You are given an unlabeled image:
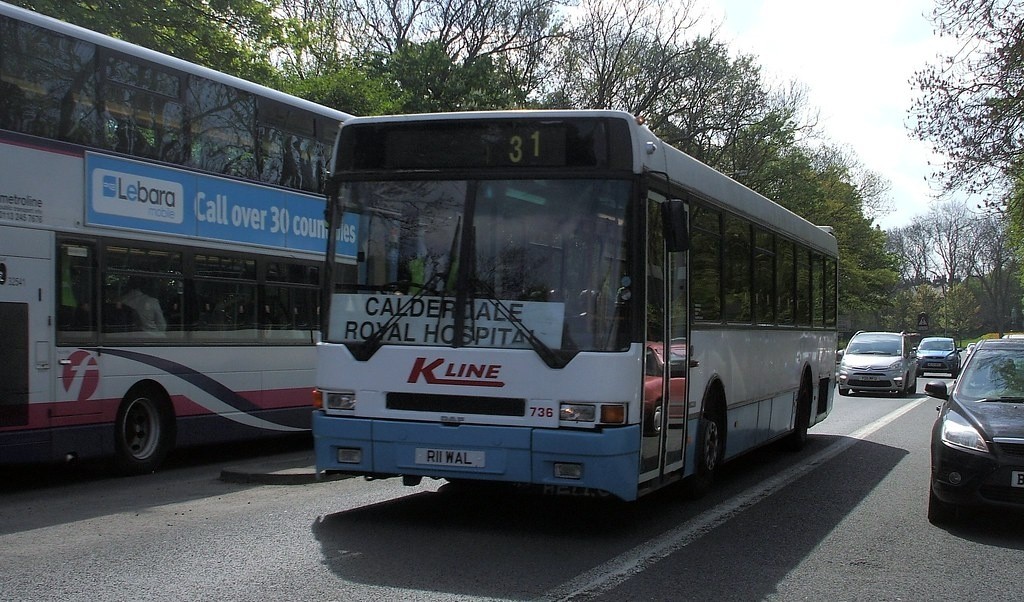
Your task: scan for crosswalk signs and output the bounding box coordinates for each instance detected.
[918,314,929,326]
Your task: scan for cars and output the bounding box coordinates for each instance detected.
[642,341,689,431]
[925,338,1024,527]
[836,331,919,394]
[914,338,961,378]
[965,342,975,355]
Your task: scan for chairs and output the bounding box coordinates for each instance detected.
[102,302,319,341]
[0,83,314,193]
[743,294,805,327]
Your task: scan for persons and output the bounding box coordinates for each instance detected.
[61,247,88,329]
[115,119,180,163]
[394,224,459,298]
[35,83,78,140]
[119,275,167,331]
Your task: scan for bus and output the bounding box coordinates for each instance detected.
[0,0,621,479]
[311,106,839,502]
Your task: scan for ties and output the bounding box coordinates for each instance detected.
[429,262,440,287]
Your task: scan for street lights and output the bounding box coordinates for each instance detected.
[934,281,947,336]
